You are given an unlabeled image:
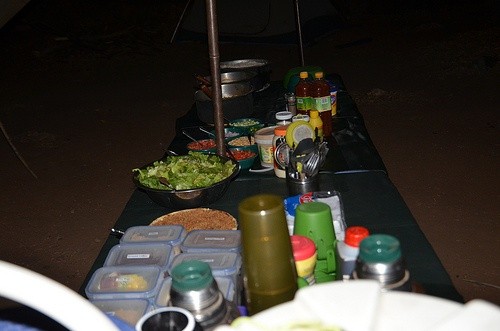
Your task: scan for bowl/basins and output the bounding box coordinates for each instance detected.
[131,152,241,208]
[185,118,262,169]
[86,226,243,326]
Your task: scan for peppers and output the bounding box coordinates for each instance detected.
[188,139,256,160]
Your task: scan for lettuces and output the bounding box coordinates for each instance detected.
[131,151,237,190]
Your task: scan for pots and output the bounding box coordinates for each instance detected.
[195,59,271,124]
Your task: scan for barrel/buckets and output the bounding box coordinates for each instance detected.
[254,126,278,168]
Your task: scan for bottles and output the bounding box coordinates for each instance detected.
[310,110,323,138]
[338,227,369,281]
[273,127,291,178]
[287,90,296,115]
[313,72,332,135]
[275,112,293,127]
[296,72,313,115]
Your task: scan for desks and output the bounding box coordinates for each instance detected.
[76,73,467,331]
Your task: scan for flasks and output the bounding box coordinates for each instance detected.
[166,260,240,331]
[350,235,414,292]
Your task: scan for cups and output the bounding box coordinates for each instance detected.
[238,194,296,295]
[329,90,337,117]
[292,203,337,283]
[288,173,319,196]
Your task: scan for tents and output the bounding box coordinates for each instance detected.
[169,0,342,49]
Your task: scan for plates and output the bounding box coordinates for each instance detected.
[148,208,238,230]
